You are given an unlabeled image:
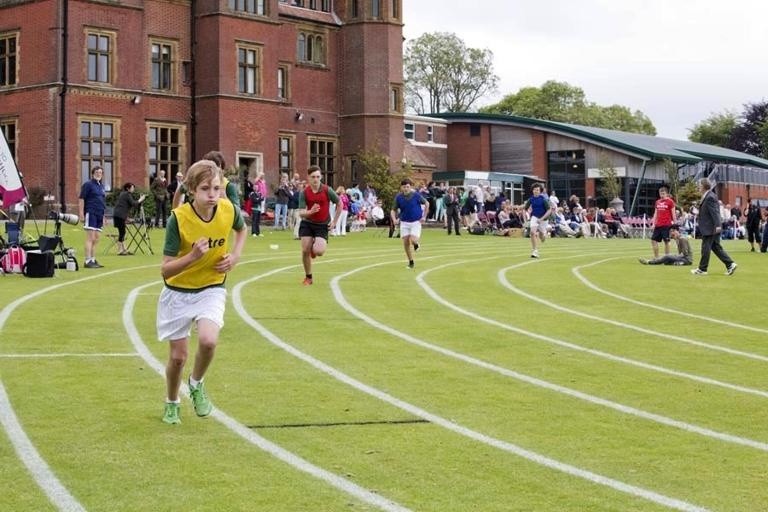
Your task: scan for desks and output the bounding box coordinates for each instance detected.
[125,222,154,255]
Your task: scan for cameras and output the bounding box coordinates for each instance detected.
[48,210,78,225]
[139,193,149,200]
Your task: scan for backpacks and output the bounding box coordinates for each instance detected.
[2,244,27,273]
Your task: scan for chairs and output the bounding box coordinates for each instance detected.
[476,210,655,235]
[103,220,119,255]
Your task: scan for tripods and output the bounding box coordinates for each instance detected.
[125,203,153,256]
[52,221,67,274]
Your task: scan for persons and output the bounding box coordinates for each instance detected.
[166,173,187,204]
[157,159,248,424]
[113,182,145,254]
[150,171,168,228]
[9,172,29,235]
[638,175,767,276]
[390,178,631,271]
[242,165,394,285]
[172,151,239,209]
[79,166,109,268]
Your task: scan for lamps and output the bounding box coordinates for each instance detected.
[131,96,141,104]
[295,113,303,119]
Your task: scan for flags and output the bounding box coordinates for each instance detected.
[0,127,27,211]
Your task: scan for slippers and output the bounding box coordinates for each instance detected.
[116,249,134,255]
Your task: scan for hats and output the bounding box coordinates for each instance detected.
[176,172,183,177]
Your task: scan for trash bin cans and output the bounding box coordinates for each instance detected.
[7,223,19,244]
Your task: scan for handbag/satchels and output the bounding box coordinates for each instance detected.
[22,250,54,278]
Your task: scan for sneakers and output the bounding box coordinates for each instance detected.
[414,243,419,252]
[690,268,707,275]
[303,277,312,286]
[408,260,414,268]
[92,260,104,268]
[638,255,649,265]
[188,374,212,416]
[84,260,99,268]
[162,397,180,424]
[531,249,538,259]
[724,263,737,276]
[310,252,316,258]
